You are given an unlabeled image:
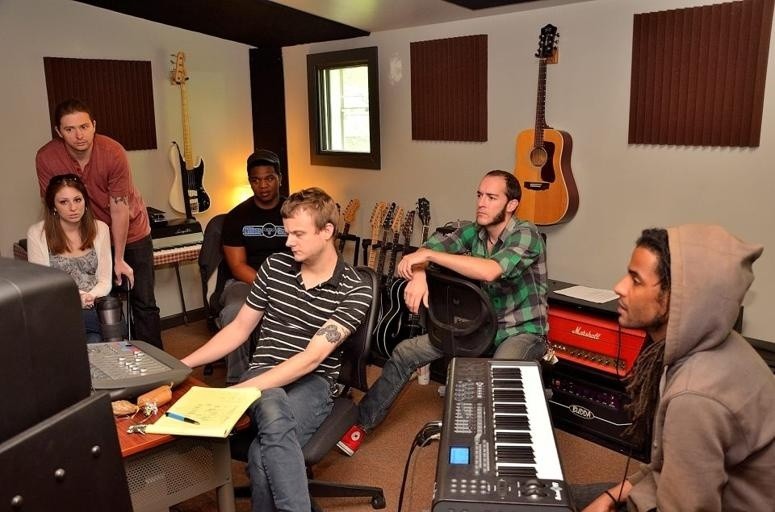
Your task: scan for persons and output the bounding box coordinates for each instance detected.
[181,187,372,510]
[578,223,775,512]
[27,98,161,353]
[218,150,295,431]
[335,170,549,457]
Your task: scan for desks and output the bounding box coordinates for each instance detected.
[112,375,251,512]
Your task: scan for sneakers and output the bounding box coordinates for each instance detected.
[336,423,366,458]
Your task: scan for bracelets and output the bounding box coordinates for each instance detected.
[603,488,619,506]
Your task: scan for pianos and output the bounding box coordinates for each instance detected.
[431,358,576,512]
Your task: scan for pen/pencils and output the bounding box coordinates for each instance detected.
[165,413,200,426]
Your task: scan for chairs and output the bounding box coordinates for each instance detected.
[414,268,498,446]
[112,247,137,340]
[232,266,386,512]
[198,213,233,376]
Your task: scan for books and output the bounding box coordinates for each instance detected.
[144,385,262,438]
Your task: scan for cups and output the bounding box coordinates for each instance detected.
[94,295,124,344]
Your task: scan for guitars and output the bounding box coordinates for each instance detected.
[338,197,431,360]
[513,24,579,226]
[169,50,210,214]
